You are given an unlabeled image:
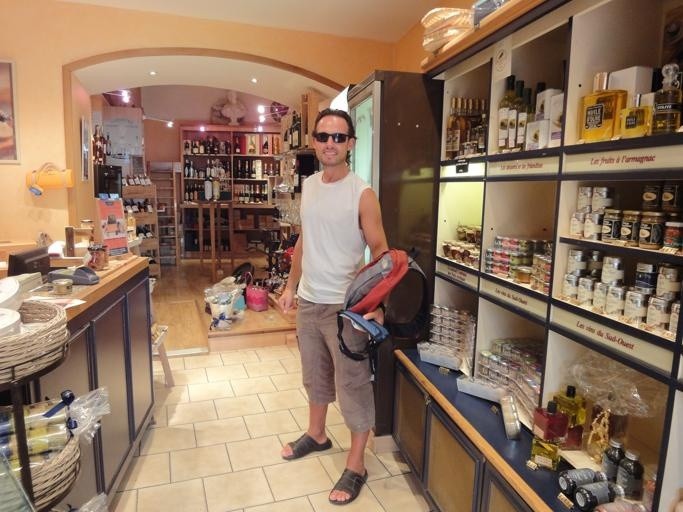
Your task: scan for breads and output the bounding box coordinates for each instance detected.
[0,401,73,432]
[0,425,74,454]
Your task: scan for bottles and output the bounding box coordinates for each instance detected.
[445,92,484,157]
[121,174,152,185]
[183,136,231,154]
[212,176,221,201]
[185,157,231,177]
[205,175,212,202]
[234,136,240,153]
[185,181,205,202]
[235,159,279,179]
[136,225,154,239]
[262,134,269,155]
[247,135,256,154]
[234,183,267,205]
[124,199,154,213]
[494,74,546,152]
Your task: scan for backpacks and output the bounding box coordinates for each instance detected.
[342,237,433,346]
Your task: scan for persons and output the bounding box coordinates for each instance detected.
[277,110,393,505]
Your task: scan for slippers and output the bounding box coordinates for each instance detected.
[329,466,368,506]
[282,435,332,461]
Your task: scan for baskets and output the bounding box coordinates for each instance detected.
[0,433,82,509]
[0,298,69,387]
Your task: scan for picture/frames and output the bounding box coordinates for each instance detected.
[0,60,22,165]
[80,115,90,182]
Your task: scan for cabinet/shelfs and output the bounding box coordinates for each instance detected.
[30,323,102,512]
[122,183,162,279]
[431,0,683,512]
[319,69,444,438]
[0,298,83,512]
[179,124,285,259]
[390,363,534,511]
[90,276,154,496]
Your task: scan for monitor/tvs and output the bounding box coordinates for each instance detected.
[93,164,122,197]
[7,246,51,276]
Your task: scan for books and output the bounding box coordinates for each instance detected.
[96,198,128,249]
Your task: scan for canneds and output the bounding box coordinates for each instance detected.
[430,298,477,370]
[601,209,619,243]
[663,221,682,250]
[639,212,664,249]
[486,235,550,294]
[441,222,480,268]
[620,209,641,247]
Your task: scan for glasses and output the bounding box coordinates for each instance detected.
[311,130,357,145]
